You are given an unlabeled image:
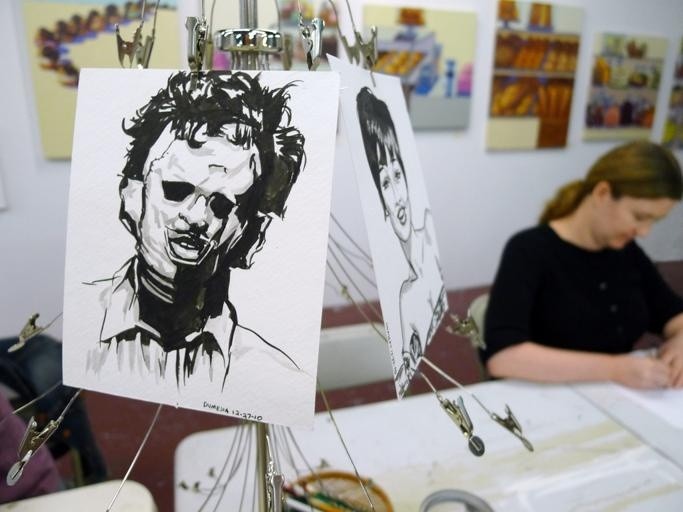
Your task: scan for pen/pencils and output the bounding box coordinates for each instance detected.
[281,481,375,512]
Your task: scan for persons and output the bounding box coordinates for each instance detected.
[357,86,450,359]
[481,139,683,392]
[85,73,301,410]
